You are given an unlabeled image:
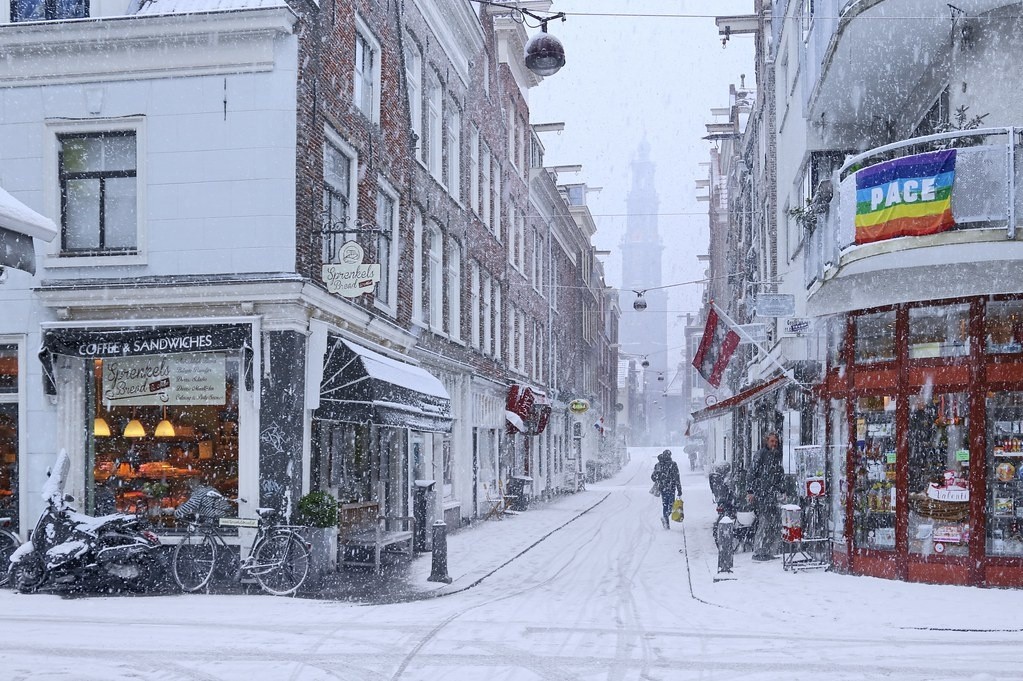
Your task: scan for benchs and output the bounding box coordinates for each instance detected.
[483,478,519,522]
[338,498,417,575]
[564,471,586,494]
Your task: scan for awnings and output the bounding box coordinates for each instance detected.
[686,429,706,439]
[509,385,551,435]
[314,336,452,434]
[691,375,794,423]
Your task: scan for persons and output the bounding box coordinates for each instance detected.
[100,476,122,515]
[649,450,682,529]
[174,475,233,527]
[688,452,697,471]
[747,431,783,561]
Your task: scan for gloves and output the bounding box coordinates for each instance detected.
[677,491,682,495]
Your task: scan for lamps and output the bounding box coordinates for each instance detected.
[94,374,111,437]
[632,289,647,312]
[123,406,146,437]
[522,7,567,76]
[641,354,649,368]
[154,405,176,437]
[657,371,664,383]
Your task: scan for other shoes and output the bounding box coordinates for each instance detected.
[764,554,780,560]
[661,516,670,529]
[752,555,767,561]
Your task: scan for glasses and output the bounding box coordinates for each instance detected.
[769,432,779,437]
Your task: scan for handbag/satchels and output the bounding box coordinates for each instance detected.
[649,482,659,497]
[672,496,684,522]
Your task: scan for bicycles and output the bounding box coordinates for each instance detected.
[711,491,756,553]
[1,518,22,587]
[172,478,313,598]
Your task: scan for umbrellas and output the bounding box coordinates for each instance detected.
[683,444,700,453]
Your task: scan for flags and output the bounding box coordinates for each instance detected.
[594,417,605,437]
[853,148,958,244]
[692,309,740,388]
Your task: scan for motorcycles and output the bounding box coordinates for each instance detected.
[8,445,167,596]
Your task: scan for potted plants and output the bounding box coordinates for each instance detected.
[289,489,339,579]
